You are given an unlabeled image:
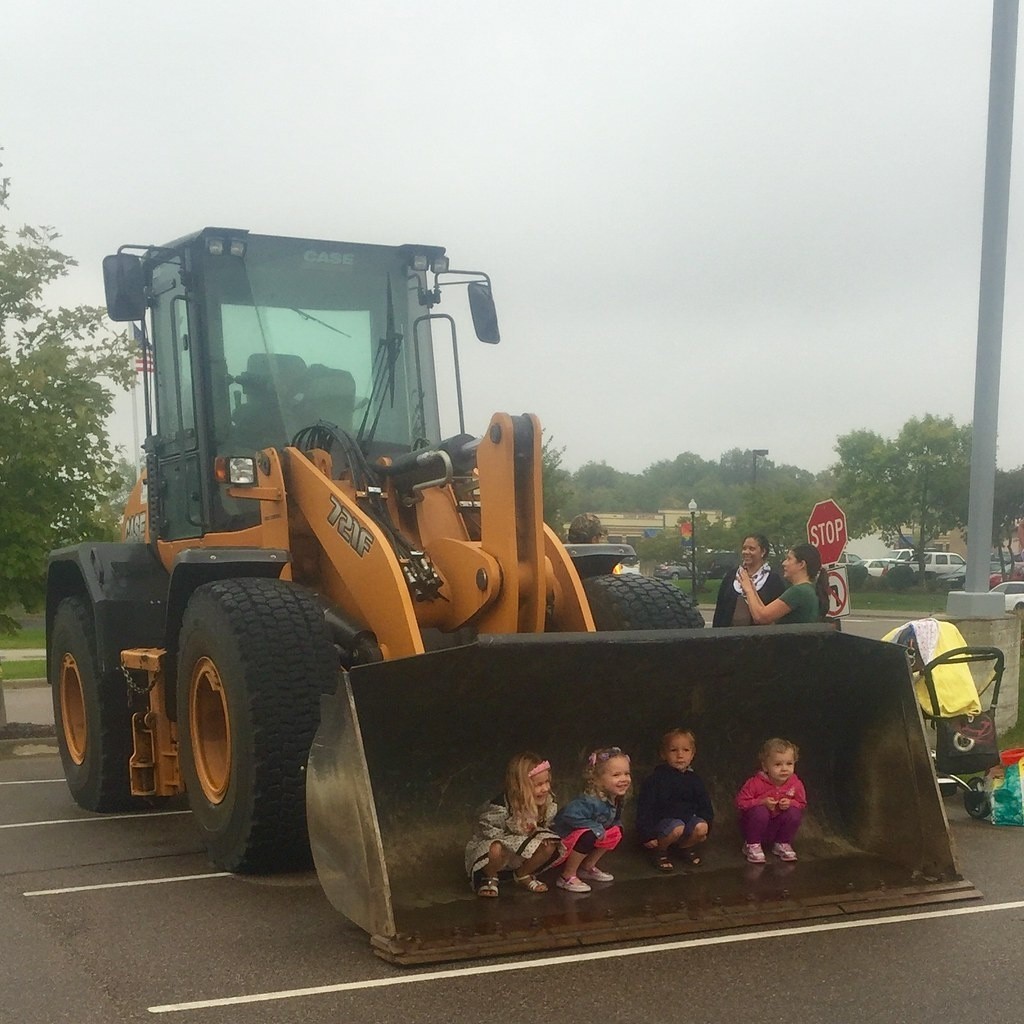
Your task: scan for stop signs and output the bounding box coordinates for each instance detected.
[805,499,849,565]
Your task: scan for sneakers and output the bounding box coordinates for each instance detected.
[742,840,766,863]
[575,865,613,881]
[556,875,590,892]
[771,841,798,861]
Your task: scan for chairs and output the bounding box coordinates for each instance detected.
[235,353,306,449]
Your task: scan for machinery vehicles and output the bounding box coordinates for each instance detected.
[41,225,986,964]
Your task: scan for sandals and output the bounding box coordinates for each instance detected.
[477,877,499,897]
[676,848,702,867]
[514,869,549,892]
[652,850,674,874]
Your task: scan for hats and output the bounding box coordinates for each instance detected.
[568,514,608,542]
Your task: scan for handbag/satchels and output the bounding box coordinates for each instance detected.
[924,656,1003,774]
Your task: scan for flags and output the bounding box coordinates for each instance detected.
[133,323,155,371]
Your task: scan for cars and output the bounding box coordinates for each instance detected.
[851,559,903,577]
[991,561,1024,590]
[653,561,698,581]
[843,554,862,565]
[991,581,1024,612]
[886,548,917,562]
[903,552,967,574]
[935,562,1007,591]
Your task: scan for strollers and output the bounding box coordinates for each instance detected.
[895,622,1005,820]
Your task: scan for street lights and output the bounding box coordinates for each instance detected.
[751,448,769,490]
[687,497,703,606]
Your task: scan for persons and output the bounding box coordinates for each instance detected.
[732,738,809,863]
[711,533,787,627]
[633,728,716,873]
[461,752,566,898]
[544,747,631,893]
[568,513,621,576]
[736,543,830,623]
